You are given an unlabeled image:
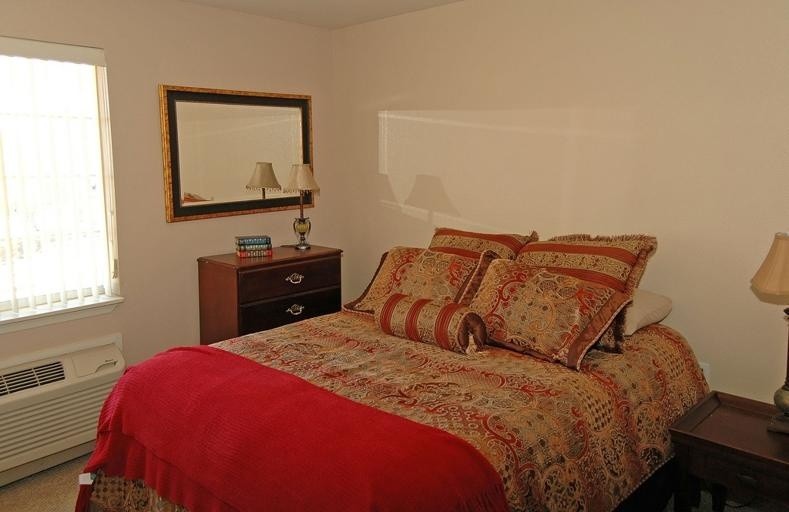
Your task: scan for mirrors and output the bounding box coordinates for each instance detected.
[158,84,314,223]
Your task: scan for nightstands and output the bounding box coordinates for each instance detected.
[667,391,789,512]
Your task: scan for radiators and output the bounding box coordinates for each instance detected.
[0,329,127,490]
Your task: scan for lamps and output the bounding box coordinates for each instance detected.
[284,157,321,251]
[749,232,789,442]
[247,161,282,199]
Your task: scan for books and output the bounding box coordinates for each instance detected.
[236,257,272,266]
[234,235,273,257]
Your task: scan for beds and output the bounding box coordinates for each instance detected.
[76,305,711,512]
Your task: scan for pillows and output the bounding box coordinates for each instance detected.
[345,248,493,321]
[433,230,541,263]
[626,290,675,338]
[378,292,489,357]
[456,249,636,372]
[515,236,657,355]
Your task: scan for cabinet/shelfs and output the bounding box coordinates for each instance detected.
[197,244,345,345]
